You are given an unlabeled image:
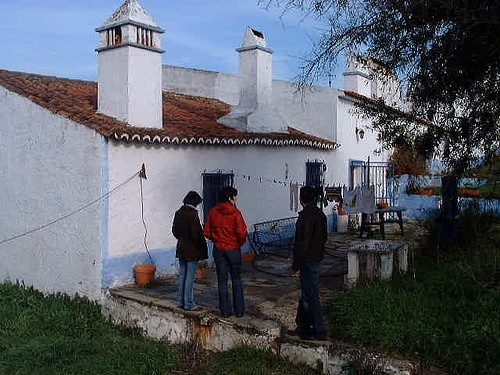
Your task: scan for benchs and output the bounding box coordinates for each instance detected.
[252,216,299,258]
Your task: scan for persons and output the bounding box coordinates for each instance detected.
[203,186,248,318]
[172,191,208,311]
[287,186,328,341]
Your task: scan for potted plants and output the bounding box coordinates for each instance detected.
[195,262,209,279]
[458,183,480,197]
[405,180,433,196]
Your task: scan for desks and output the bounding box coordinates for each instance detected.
[359,206,406,240]
[345,240,407,286]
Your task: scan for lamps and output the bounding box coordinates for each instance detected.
[355,127,365,139]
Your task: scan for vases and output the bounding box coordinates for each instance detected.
[135,262,156,286]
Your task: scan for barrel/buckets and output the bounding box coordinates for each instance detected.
[134,263,156,288]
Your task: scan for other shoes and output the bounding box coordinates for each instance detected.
[221,311,233,318]
[237,311,244,318]
[302,334,327,341]
[287,328,299,336]
[184,304,203,311]
